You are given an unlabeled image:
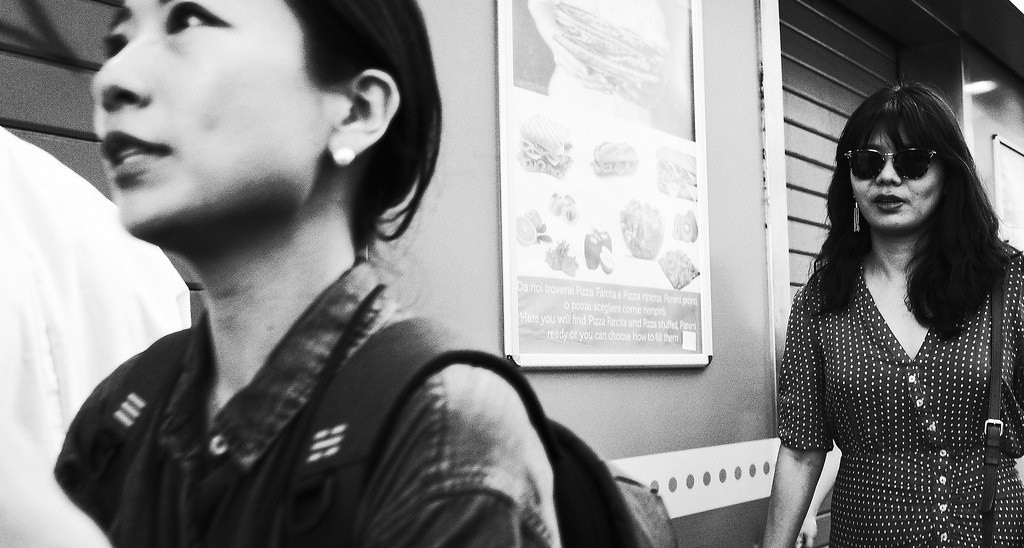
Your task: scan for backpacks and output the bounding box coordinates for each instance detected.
[80,314,679,547]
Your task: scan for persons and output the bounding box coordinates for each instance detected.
[761,84,1024,548]
[0,0,563,547]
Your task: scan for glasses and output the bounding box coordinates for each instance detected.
[843,148,938,180]
